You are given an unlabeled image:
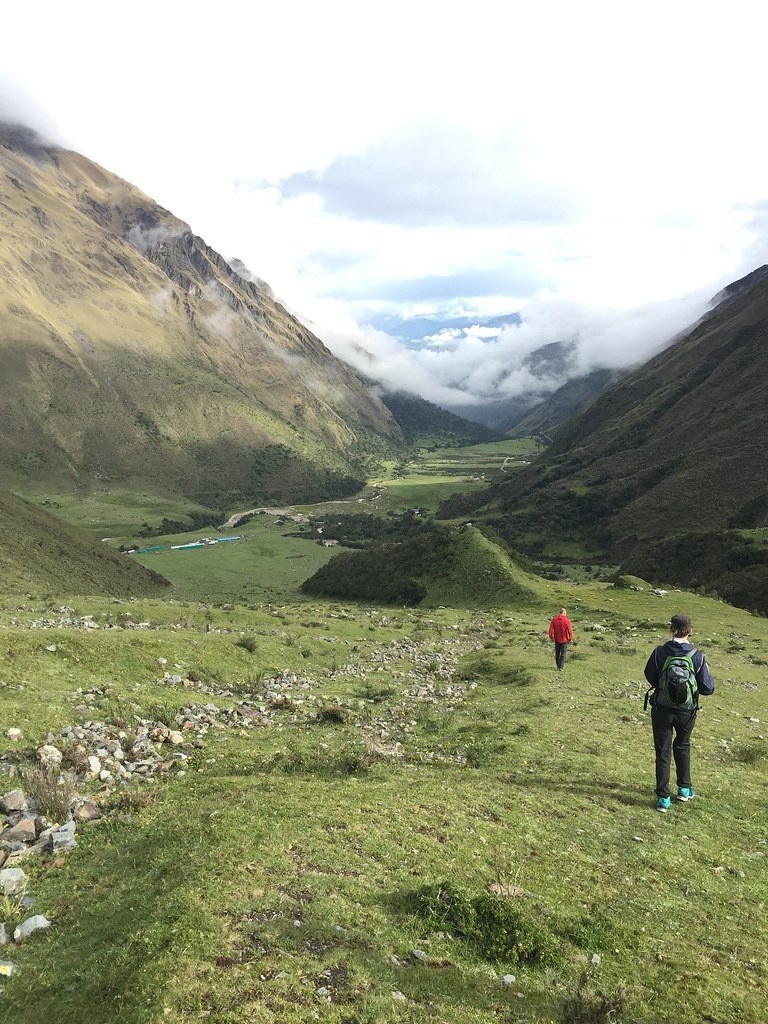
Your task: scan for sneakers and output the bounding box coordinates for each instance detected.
[656,796,671,812]
[677,787,694,801]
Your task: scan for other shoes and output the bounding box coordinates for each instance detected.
[559,668,563,671]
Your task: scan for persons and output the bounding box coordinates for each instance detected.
[644,615,715,813]
[549,608,572,672]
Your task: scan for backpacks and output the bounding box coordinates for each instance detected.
[654,646,698,711]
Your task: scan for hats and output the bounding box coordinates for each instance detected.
[671,614,692,630]
